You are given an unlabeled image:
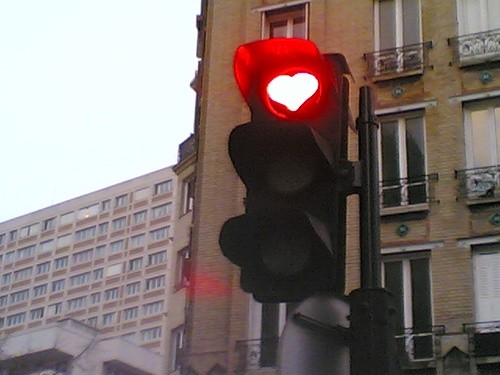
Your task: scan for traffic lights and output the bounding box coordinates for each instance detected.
[219,37,354,302]
